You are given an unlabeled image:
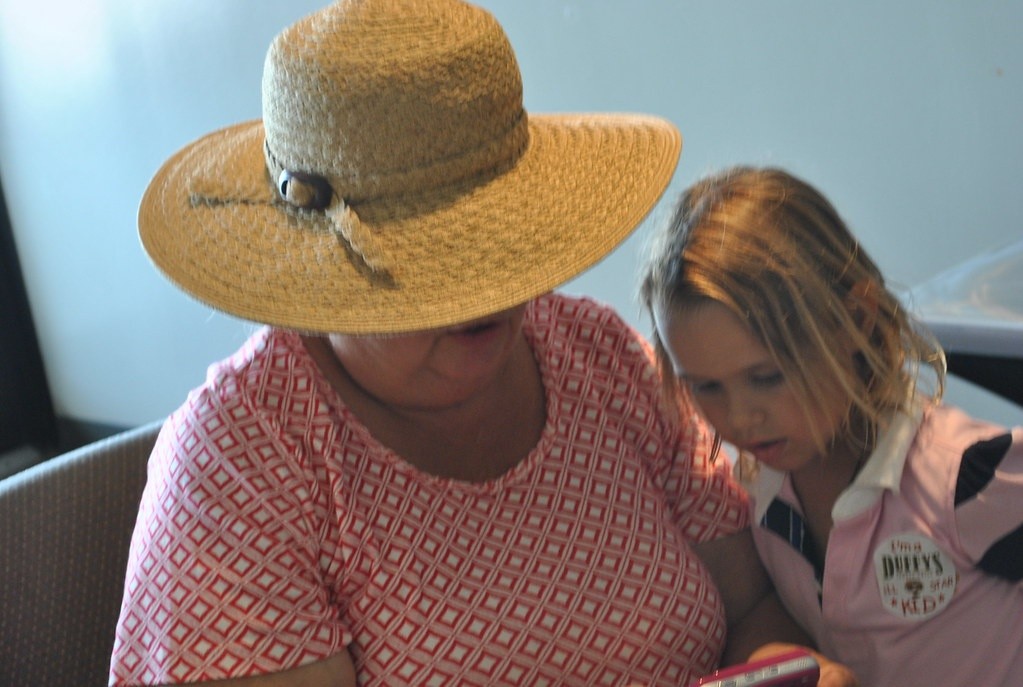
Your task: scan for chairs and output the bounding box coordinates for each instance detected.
[0,419,164,687]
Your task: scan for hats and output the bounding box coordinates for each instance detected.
[137,0,683,335]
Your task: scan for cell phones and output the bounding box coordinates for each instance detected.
[691,650,820,687]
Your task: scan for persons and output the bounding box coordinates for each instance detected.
[641,164,1023,687]
[106,1,855,687]
